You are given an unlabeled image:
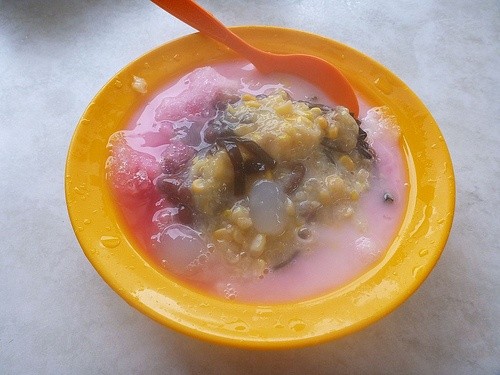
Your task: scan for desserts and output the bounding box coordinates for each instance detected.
[106,56,410,306]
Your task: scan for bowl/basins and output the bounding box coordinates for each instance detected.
[63,25,457,347]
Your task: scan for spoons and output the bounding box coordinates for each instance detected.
[149,0,361,121]
